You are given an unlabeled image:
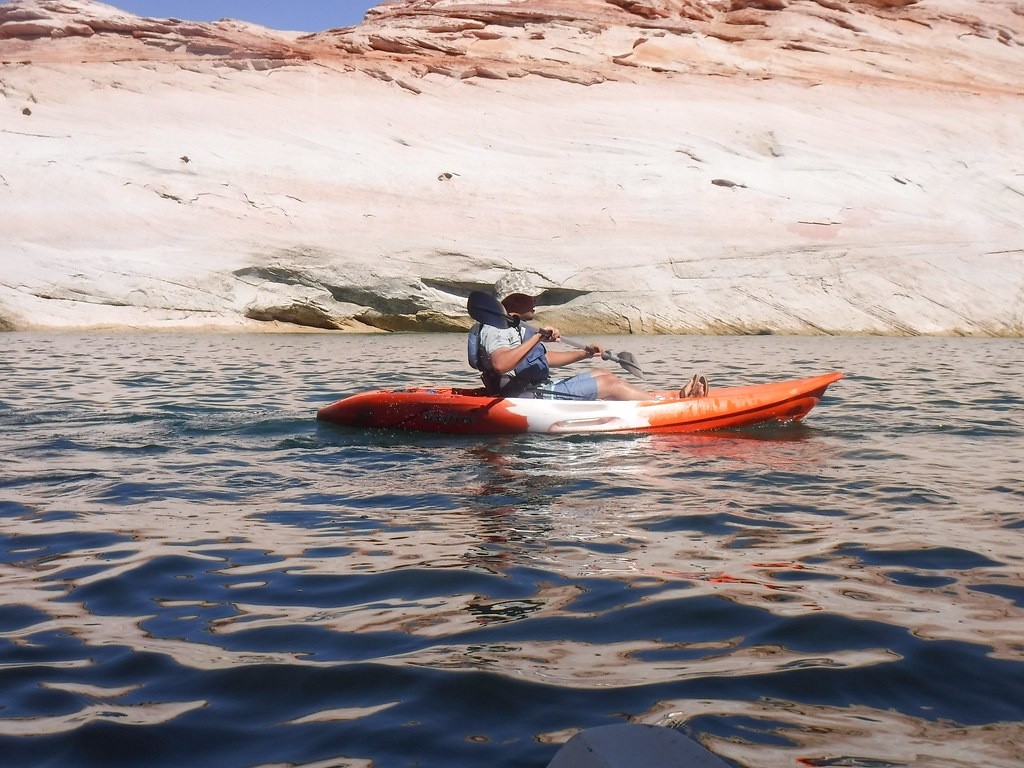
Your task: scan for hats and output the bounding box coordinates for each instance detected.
[494,271,548,302]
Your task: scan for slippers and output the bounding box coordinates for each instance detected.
[688,375,709,398]
[679,374,699,398]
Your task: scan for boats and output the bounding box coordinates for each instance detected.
[314,371,845,434]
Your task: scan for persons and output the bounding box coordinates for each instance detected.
[478,272,709,401]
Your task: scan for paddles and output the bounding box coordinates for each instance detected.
[467,290,645,382]
